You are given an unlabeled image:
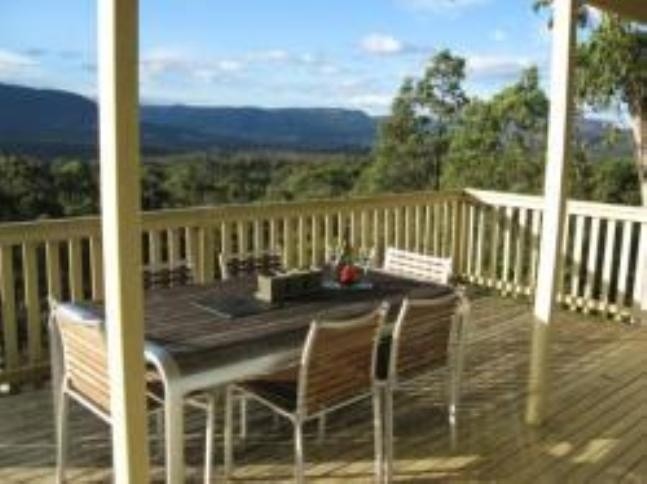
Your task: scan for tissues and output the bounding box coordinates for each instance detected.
[257,266,325,301]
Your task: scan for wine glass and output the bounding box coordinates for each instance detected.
[359,247,375,284]
